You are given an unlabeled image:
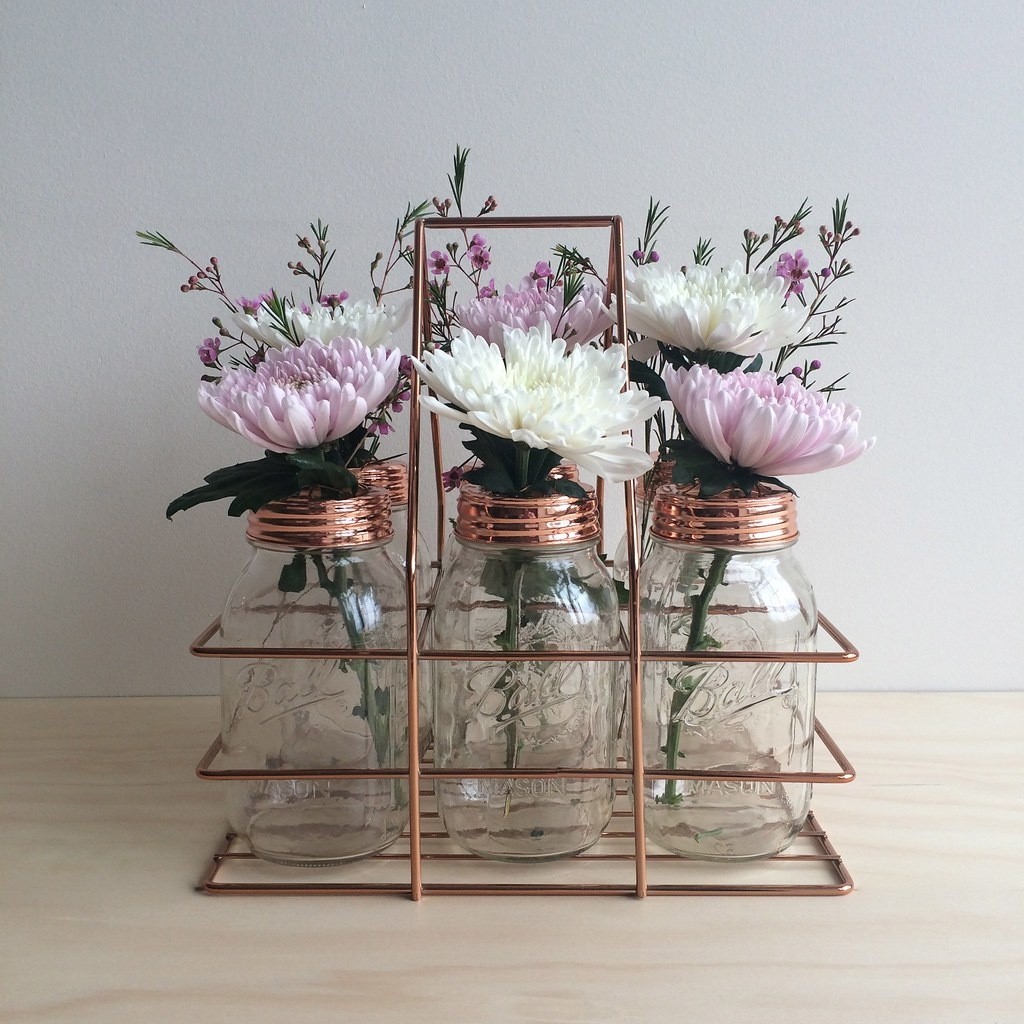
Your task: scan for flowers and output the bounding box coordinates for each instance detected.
[135,141,876,805]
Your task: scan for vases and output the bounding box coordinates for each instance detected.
[208,450,825,866]
